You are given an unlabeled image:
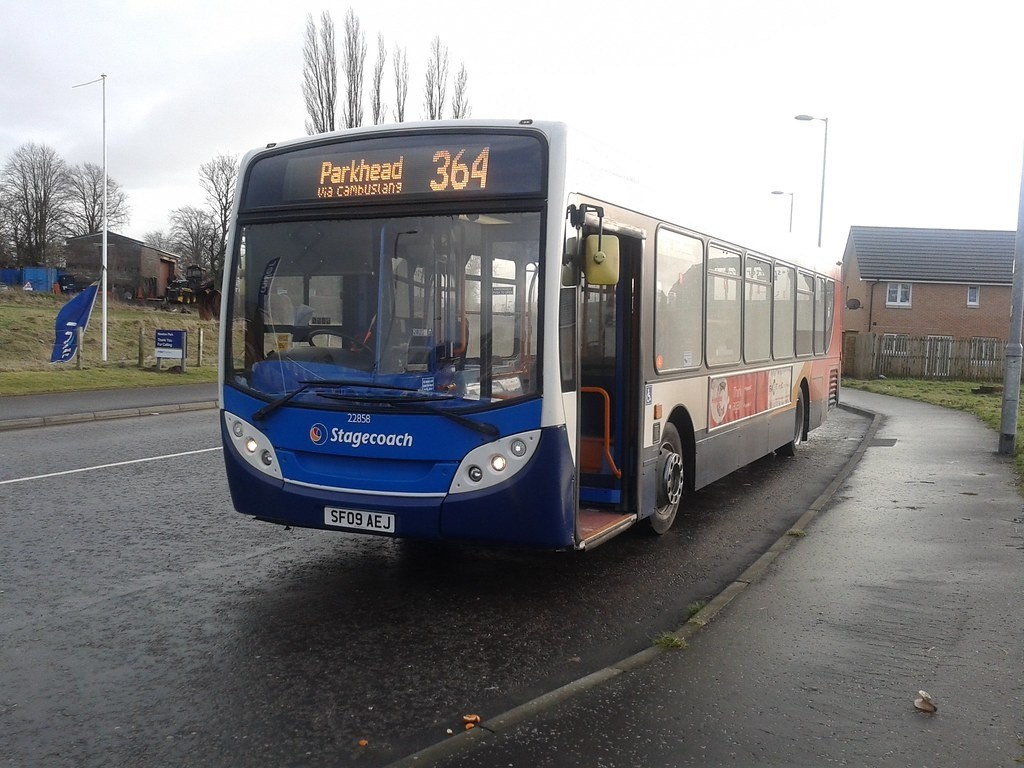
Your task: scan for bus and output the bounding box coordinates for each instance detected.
[217,118,845,556]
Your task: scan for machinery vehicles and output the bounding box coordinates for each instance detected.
[167,264,207,305]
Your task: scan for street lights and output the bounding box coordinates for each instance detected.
[794,114,828,249]
[771,190,794,233]
[394,229,418,290]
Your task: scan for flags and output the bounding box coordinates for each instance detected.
[49,278,101,365]
